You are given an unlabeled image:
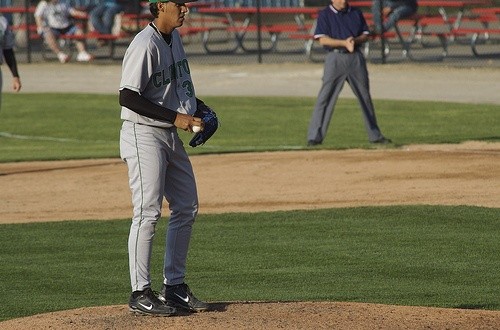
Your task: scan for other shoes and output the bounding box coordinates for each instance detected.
[372,137,392,143]
[57,51,69,64]
[76,50,94,62]
[306,139,321,146]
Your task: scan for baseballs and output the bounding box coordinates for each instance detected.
[191,125,202,133]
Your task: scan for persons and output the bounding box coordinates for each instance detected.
[307,0,420,148]
[34,0,129,64]
[0,10,21,108]
[119,0,215,316]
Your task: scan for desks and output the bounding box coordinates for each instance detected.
[0,7,42,13]
[196,7,327,55]
[348,1,491,42]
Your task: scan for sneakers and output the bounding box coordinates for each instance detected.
[158,281,210,313]
[127,287,177,317]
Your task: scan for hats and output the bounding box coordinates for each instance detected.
[149,0,198,4]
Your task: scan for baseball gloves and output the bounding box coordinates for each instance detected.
[184,97,220,149]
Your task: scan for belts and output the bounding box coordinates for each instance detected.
[331,48,349,53]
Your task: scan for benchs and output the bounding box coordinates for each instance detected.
[10,17,500,62]
